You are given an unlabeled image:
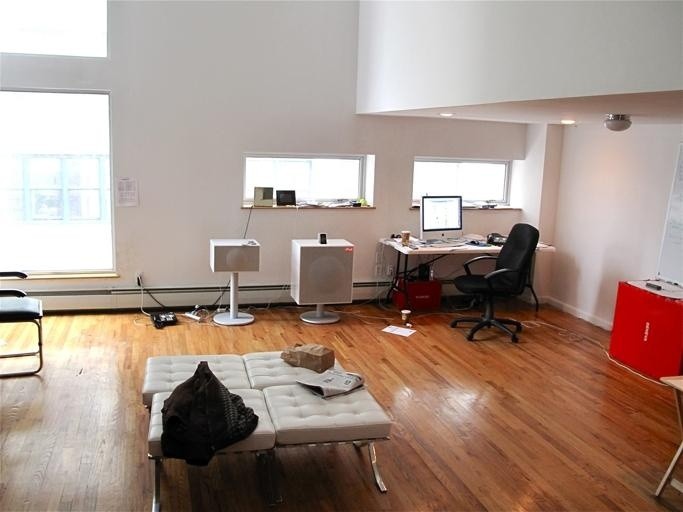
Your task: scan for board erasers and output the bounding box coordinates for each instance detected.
[646,283,662,291]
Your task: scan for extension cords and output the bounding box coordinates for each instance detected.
[184,312,203,322]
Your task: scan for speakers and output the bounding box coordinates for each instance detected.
[290,233,355,306]
[209,237,261,273]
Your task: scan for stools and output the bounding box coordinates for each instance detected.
[264,384,392,501]
[144,354,250,390]
[244,350,347,389]
[149,392,275,511]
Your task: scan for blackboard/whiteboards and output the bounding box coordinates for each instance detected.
[656,143,683,289]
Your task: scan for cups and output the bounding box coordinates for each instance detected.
[402,231,410,247]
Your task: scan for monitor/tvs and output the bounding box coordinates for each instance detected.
[420,195,465,247]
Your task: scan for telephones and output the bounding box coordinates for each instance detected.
[487,232,508,245]
[150,310,177,328]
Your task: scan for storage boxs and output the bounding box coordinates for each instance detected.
[394,278,442,312]
[611,278,683,385]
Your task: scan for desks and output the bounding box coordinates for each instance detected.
[654,376,683,501]
[379,237,556,312]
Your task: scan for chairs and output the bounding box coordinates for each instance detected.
[455,223,539,341]
[0,272,44,379]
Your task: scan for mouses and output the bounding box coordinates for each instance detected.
[470,240,479,245]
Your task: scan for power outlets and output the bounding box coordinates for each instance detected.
[135,272,145,287]
